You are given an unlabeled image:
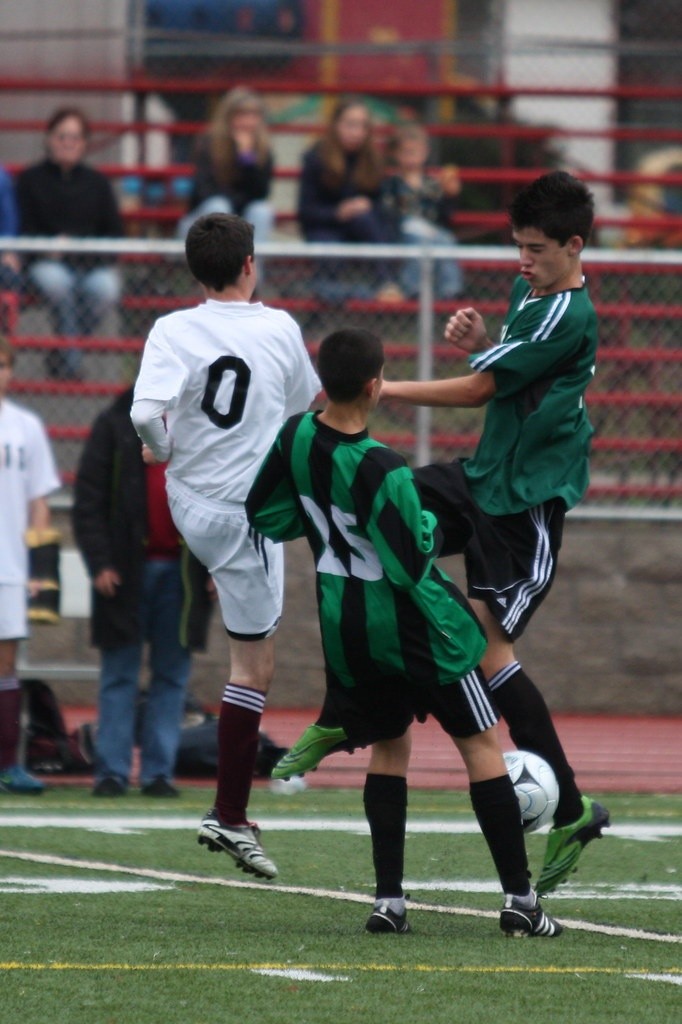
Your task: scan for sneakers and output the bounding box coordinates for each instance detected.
[197,807,279,879]
[0,766,44,794]
[500,888,564,939]
[272,724,354,781]
[534,795,610,897]
[364,891,412,935]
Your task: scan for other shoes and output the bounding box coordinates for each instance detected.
[92,778,125,796]
[141,776,180,798]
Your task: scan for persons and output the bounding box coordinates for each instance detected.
[245,331,564,936]
[70,385,217,799]
[0,338,62,796]
[0,167,23,339]
[131,211,322,880]
[270,169,612,899]
[297,100,474,303]
[177,86,274,297]
[11,106,126,384]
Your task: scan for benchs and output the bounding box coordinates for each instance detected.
[0,63,682,499]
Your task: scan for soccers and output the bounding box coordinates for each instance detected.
[501,748,562,835]
